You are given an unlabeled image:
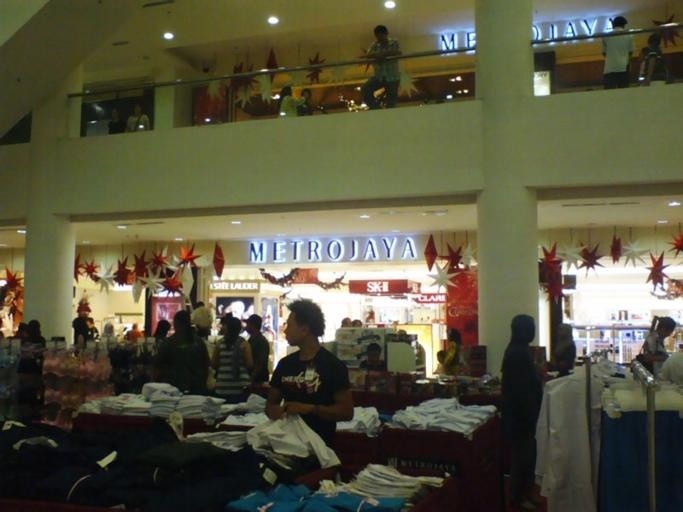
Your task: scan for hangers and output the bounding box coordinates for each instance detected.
[0,334,161,362]
[601,360,682,420]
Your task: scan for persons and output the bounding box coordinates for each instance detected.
[361,25,402,109]
[360,343,385,370]
[108,102,150,134]
[432,329,461,374]
[152,301,270,403]
[642,316,676,361]
[279,85,312,117]
[501,315,576,512]
[126,323,143,340]
[601,16,666,89]
[72,318,99,357]
[266,299,353,447]
[16,320,45,403]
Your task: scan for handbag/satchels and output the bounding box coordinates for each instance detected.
[636,334,658,374]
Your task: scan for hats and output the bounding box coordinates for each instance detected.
[243,315,263,328]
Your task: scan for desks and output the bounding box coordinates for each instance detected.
[70,373,507,512]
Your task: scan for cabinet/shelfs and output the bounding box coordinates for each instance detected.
[323,324,425,382]
